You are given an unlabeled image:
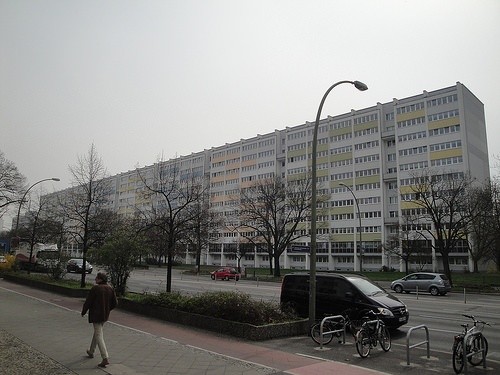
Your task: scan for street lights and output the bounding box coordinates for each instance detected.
[308,80,370,338]
[338,182,363,272]
[13,177,61,247]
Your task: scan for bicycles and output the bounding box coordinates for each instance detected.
[309,308,357,346]
[352,309,392,359]
[451,314,492,374]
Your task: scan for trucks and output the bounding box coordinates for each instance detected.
[18,241,61,273]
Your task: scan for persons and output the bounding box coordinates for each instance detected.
[81,272,118,366]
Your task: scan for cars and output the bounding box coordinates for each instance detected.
[390,272,452,297]
[209,267,242,282]
[66,259,94,274]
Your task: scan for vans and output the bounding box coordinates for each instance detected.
[279,271,410,331]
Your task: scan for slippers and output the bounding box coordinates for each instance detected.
[86,349,94,358]
[98,361,109,367]
